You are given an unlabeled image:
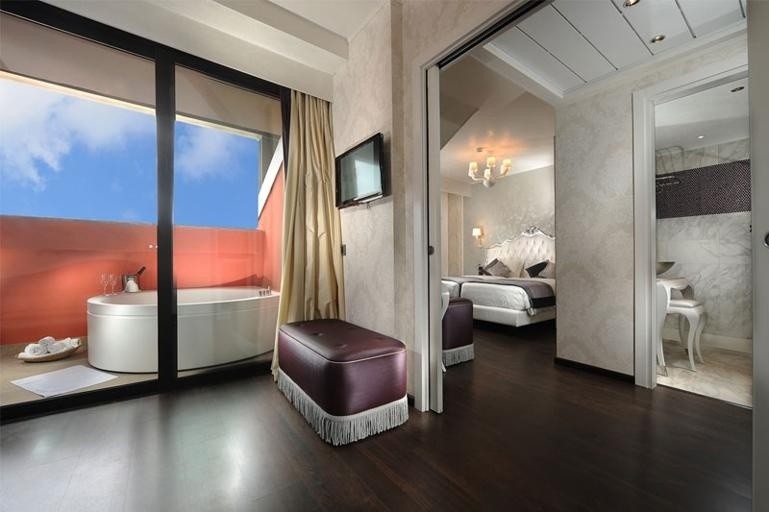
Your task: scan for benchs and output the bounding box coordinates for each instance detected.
[271,318,409,447]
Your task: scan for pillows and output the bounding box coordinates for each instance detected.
[479,253,556,277]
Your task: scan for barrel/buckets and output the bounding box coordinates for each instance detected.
[122,271,139,291]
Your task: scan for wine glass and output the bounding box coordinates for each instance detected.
[110,271,118,296]
[98,271,110,297]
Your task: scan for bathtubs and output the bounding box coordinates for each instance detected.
[87,286,280,374]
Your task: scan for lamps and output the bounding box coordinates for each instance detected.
[467,152,512,189]
[472,227,483,249]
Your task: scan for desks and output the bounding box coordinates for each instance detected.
[655,276,694,376]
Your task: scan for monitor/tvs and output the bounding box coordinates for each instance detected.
[333,132,386,208]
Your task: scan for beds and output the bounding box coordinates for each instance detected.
[440,227,557,328]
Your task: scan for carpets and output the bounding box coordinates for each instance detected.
[9,365,119,398]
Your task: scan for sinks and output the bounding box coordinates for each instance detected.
[655,260,676,275]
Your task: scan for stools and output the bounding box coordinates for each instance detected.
[666,298,708,372]
